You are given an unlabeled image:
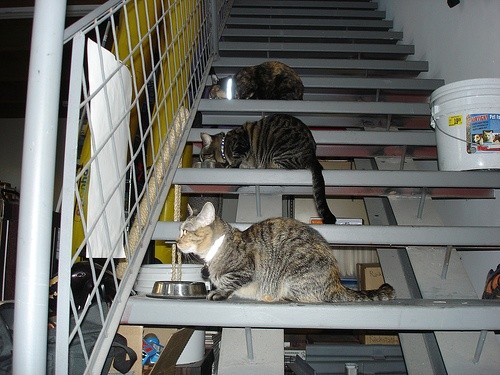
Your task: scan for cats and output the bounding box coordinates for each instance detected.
[175,202,396,303]
[200,113,336,224]
[483,130,500,143]
[209,61,305,101]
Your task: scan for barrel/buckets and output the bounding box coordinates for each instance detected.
[425,76,500,172]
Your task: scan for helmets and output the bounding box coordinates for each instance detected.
[47,261,137,315]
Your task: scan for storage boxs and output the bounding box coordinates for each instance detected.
[107,324,196,375]
[355,262,401,345]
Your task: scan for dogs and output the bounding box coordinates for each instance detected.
[473,134,484,145]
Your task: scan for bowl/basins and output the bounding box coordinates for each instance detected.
[146,280,208,299]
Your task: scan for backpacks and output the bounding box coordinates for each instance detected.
[0,302,137,375]
